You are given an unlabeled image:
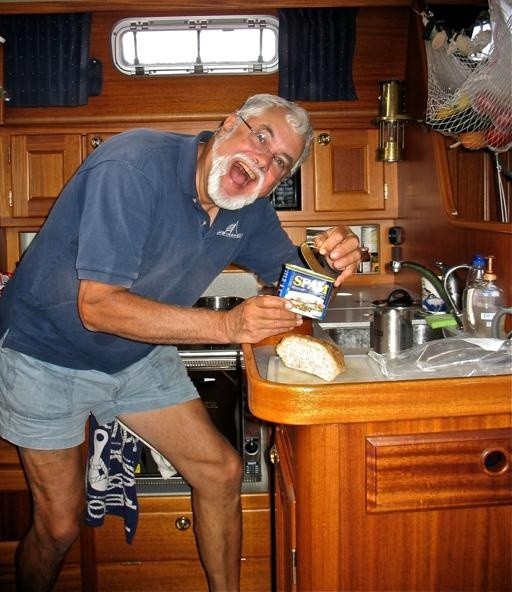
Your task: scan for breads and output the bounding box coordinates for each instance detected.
[277,335,346,381]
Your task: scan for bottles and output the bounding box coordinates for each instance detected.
[357,247,371,273]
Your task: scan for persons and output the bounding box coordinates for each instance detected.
[0,92,362,591]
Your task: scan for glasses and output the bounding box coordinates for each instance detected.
[239,115,288,172]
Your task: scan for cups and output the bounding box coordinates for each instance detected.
[375,306,415,354]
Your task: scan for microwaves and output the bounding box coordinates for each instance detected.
[134,357,269,496]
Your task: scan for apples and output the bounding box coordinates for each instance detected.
[472,91,512,147]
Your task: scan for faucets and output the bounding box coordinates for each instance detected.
[389,258,460,313]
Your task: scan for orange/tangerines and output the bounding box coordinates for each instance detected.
[462,132,485,148]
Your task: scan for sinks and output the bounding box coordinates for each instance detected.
[320,318,476,360]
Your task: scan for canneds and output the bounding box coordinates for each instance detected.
[276,264,335,321]
[357,227,379,273]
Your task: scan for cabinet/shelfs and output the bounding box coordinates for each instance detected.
[1,115,512,592]
